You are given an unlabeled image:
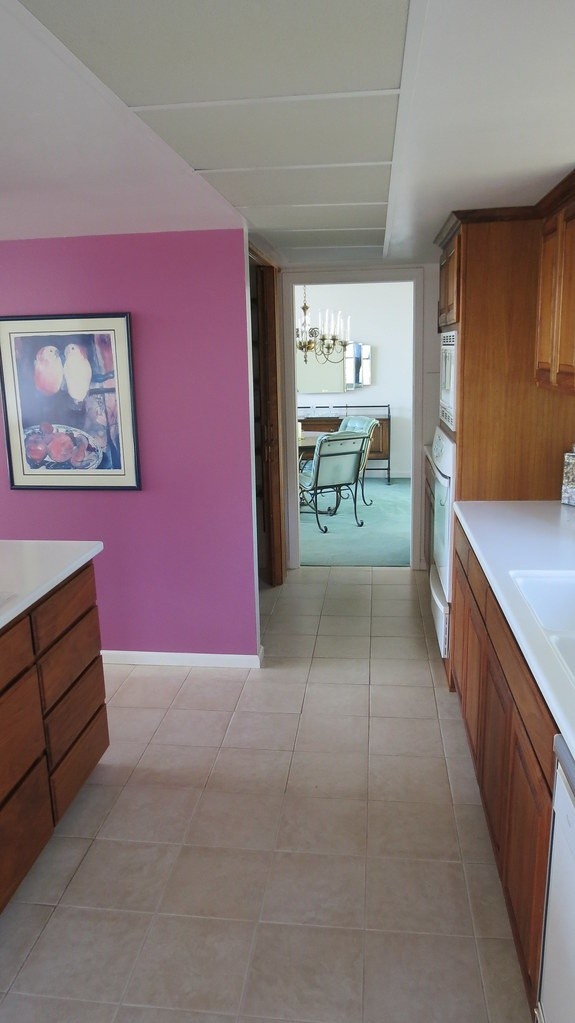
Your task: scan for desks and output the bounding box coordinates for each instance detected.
[298,430,331,513]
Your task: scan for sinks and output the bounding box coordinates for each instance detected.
[509,564,571,677]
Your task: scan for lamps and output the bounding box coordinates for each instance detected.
[296,285,350,363]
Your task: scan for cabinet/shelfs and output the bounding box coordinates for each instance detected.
[445,519,559,1023]
[0,539,110,911]
[535,203,575,398]
[437,234,460,327]
[298,406,391,484]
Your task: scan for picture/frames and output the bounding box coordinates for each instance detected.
[0,310,141,490]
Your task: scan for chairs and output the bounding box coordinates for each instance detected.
[299,430,369,533]
[328,415,380,510]
